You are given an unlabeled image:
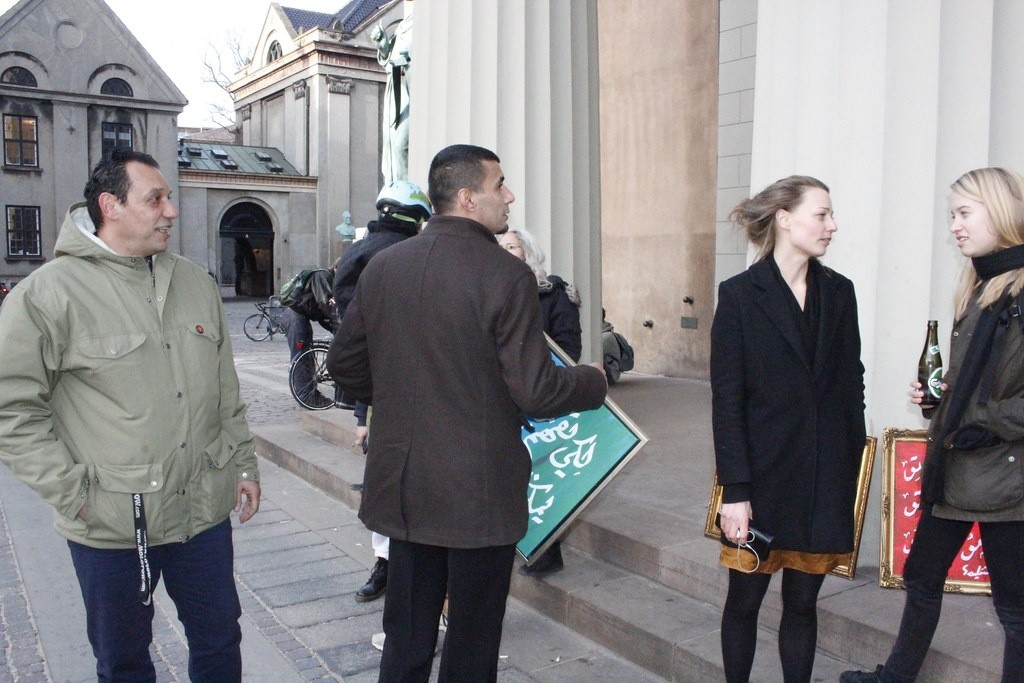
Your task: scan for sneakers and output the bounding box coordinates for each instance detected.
[439,613,449,630]
[839,664,884,683]
[372,633,439,657]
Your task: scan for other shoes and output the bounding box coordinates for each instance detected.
[300,390,333,409]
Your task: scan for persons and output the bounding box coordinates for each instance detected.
[275,252,348,408]
[371,11,414,187]
[325,145,635,683]
[839,167,1024,683]
[708,175,866,683]
[335,210,356,236]
[1,146,264,683]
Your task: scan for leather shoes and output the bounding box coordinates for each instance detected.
[355,557,389,602]
[518,541,564,577]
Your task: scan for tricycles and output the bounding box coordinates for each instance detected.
[243,295,287,342]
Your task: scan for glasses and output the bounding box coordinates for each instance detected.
[499,243,522,250]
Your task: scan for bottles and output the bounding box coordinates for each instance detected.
[917,319,942,406]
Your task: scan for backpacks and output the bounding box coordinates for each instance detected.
[278,268,334,307]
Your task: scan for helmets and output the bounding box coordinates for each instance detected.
[376,181,432,221]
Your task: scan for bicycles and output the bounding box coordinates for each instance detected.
[288,339,337,412]
